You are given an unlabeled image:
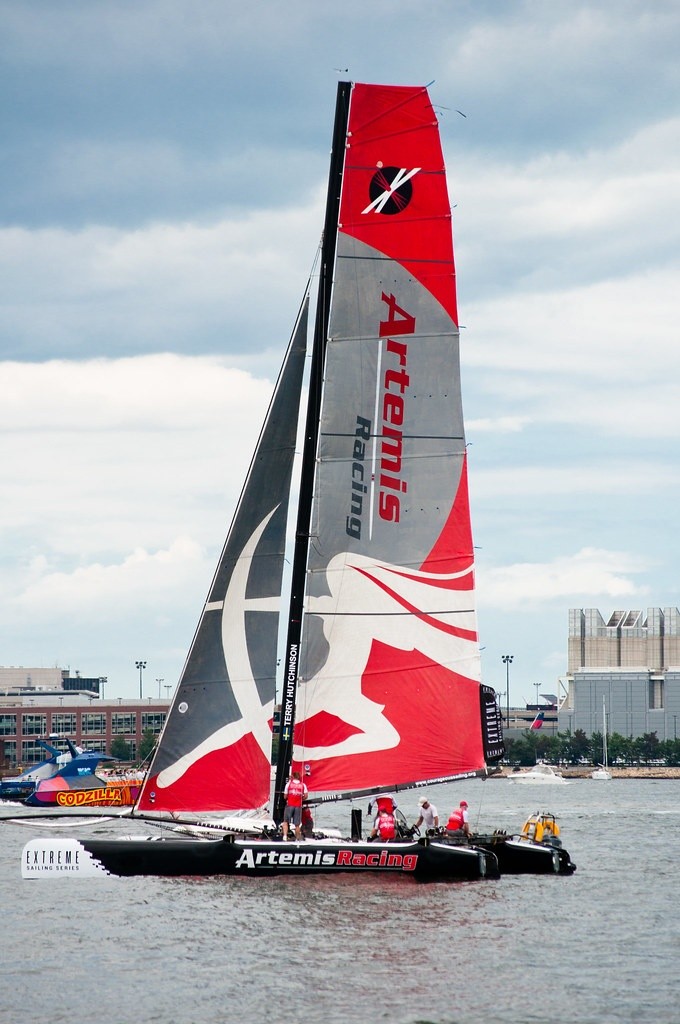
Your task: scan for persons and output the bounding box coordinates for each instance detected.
[21,775,39,787]
[283,772,308,841]
[368,793,397,837]
[76,745,83,754]
[445,801,468,836]
[373,809,396,841]
[104,769,146,780]
[416,797,438,829]
[565,764,568,769]
[301,808,313,837]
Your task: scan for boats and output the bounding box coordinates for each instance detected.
[501,831,575,876]
[23,739,149,806]
[506,761,572,787]
[1,711,84,799]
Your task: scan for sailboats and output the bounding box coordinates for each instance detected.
[74,80,501,880]
[592,692,612,780]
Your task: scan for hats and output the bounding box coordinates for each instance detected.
[417,797,427,807]
[460,801,468,807]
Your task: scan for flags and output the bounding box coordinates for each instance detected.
[531,712,545,729]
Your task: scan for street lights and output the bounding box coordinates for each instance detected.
[533,682,542,705]
[496,690,506,709]
[147,694,152,708]
[605,712,610,752]
[74,669,81,679]
[568,715,572,735]
[502,655,514,727]
[155,678,164,699]
[135,660,147,698]
[164,685,172,699]
[552,722,555,740]
[59,695,65,707]
[88,696,91,705]
[100,675,108,700]
[30,698,32,705]
[673,715,677,737]
[116,697,124,707]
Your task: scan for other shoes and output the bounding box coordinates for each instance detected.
[282,835,287,841]
[295,839,300,842]
